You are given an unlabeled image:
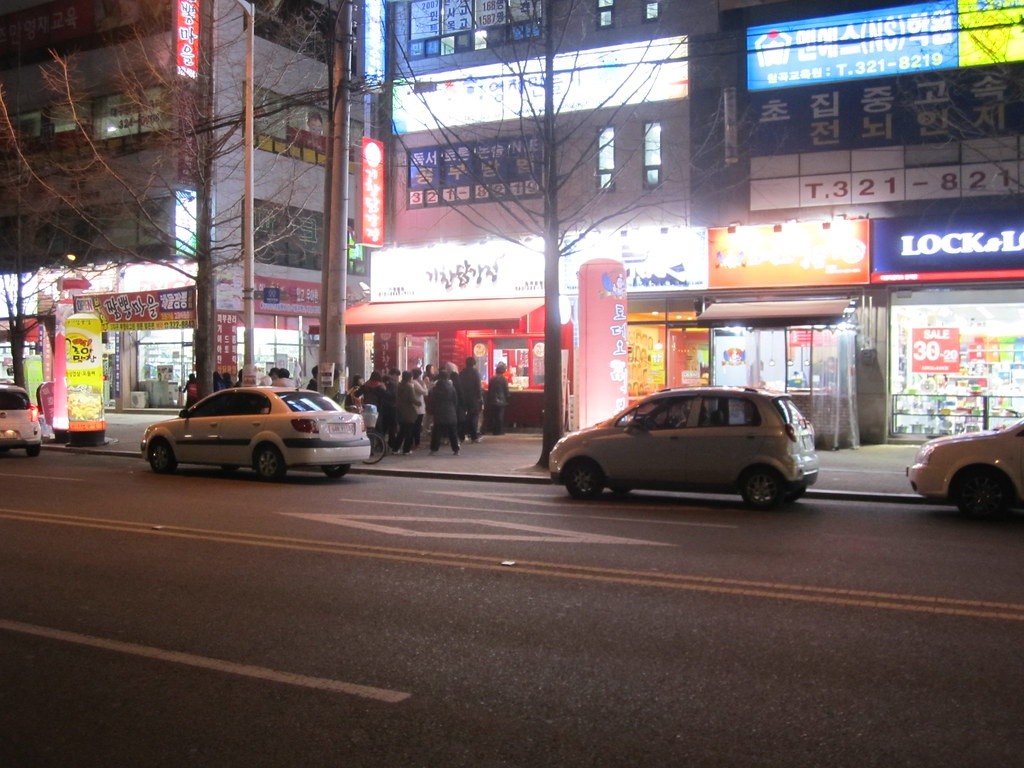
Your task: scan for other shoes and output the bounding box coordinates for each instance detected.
[428,448,439,456]
[469,437,481,443]
[453,450,460,455]
[402,450,412,456]
[392,450,401,455]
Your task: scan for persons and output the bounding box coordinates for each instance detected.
[307,112,323,135]
[213,369,243,391]
[819,357,834,384]
[306,364,318,391]
[346,357,486,456]
[183,374,198,408]
[676,404,705,428]
[488,362,512,434]
[260,367,296,387]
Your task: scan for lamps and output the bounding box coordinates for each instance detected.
[621,231,626,237]
[823,223,831,230]
[660,227,668,233]
[773,223,782,232]
[728,224,736,233]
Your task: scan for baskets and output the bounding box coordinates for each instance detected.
[361,411,379,428]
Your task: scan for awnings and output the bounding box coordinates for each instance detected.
[695,299,850,326]
[307,298,544,335]
[0,318,39,343]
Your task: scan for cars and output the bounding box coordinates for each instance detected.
[141,385,370,480]
[0,382,42,459]
[907,416,1024,521]
[548,386,819,510]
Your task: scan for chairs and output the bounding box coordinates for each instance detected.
[698,408,724,425]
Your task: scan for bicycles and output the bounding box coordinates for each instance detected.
[344,403,387,465]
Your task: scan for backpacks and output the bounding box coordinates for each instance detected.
[187,379,198,399]
[214,380,226,392]
